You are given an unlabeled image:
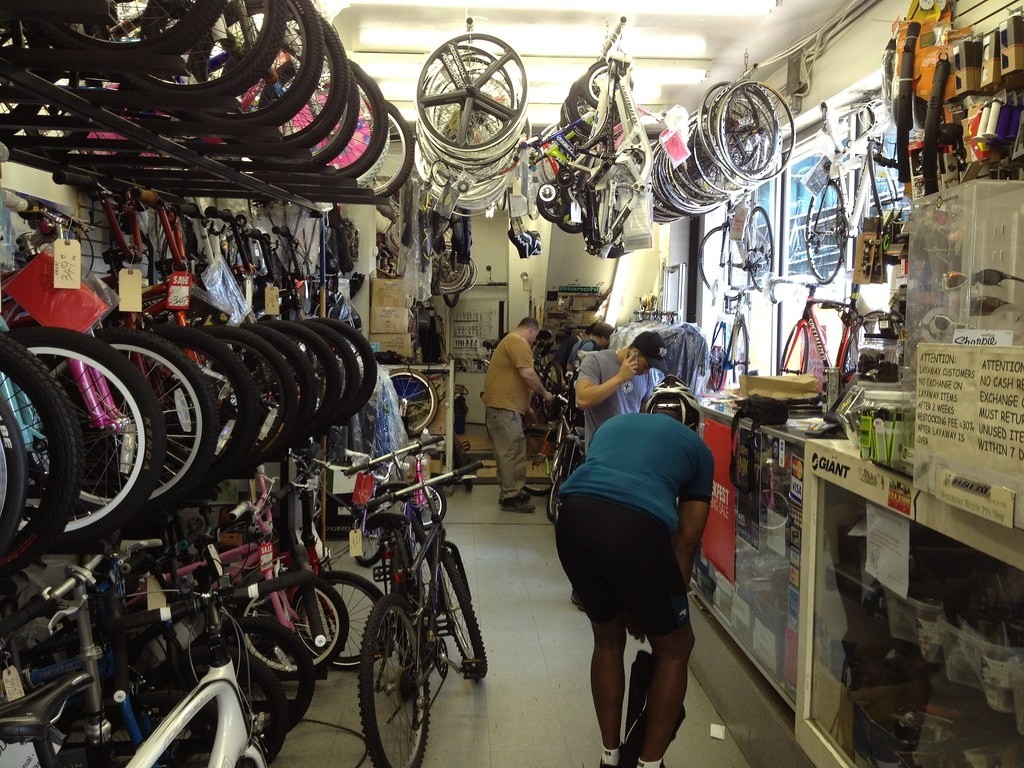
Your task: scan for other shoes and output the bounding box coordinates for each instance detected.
[600,742,624,768]
[501,496,535,512]
[499,493,531,504]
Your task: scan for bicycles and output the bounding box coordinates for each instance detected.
[696,186,775,298]
[482,330,586,523]
[709,289,750,392]
[537,60,690,259]
[1,0,489,768]
[776,277,900,385]
[807,99,909,285]
[742,443,789,528]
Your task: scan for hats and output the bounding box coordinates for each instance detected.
[634,331,668,374]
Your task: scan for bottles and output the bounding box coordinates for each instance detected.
[821,367,841,417]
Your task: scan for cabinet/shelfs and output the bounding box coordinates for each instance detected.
[380,359,457,476]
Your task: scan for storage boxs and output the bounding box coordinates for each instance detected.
[430,455,442,476]
[902,179,1024,526]
[716,548,1011,767]
[371,279,415,362]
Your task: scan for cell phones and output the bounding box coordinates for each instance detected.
[628,351,638,362]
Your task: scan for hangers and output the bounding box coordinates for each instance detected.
[631,310,687,328]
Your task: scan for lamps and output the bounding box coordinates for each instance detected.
[520,271,529,281]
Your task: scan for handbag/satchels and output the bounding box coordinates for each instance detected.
[551,397,584,427]
[625,650,685,768]
[729,394,789,494]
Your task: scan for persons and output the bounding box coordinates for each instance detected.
[481,315,554,512]
[554,375,717,768]
[575,331,669,461]
[536,319,614,428]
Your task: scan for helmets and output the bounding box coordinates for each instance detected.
[645,374,701,435]
